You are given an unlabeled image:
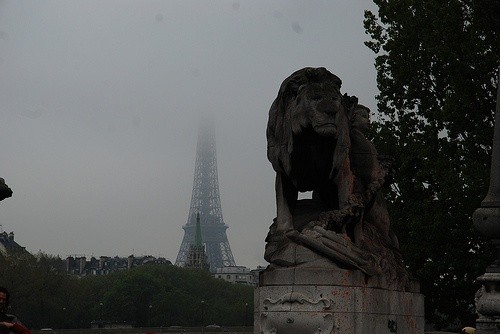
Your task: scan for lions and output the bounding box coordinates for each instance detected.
[264,67,411,292]
